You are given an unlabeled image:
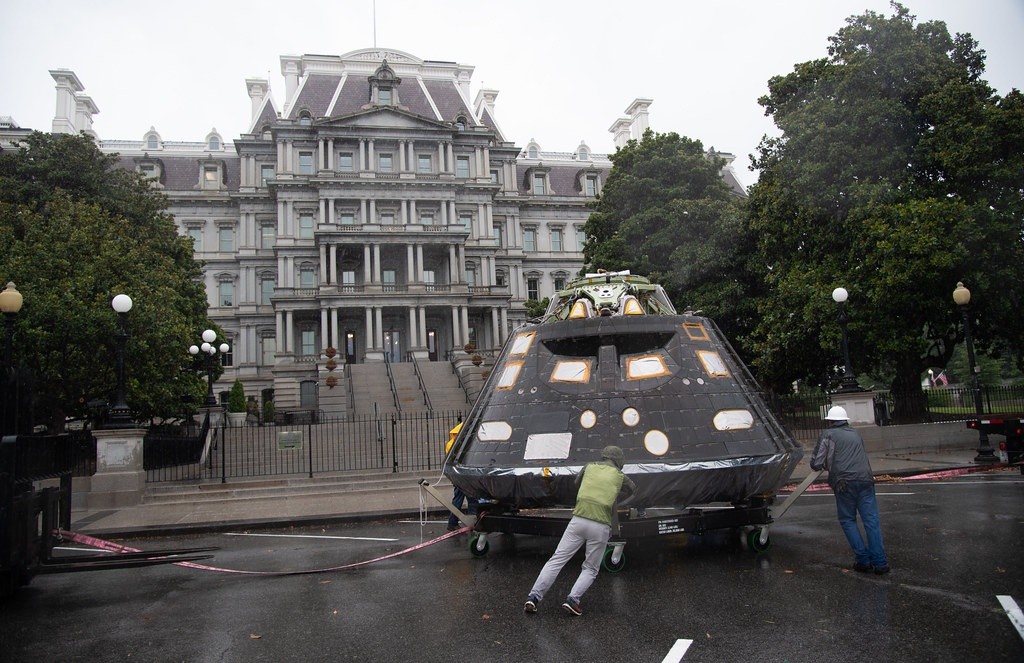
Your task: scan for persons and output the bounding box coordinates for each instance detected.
[523,445,641,615]
[809,406,893,575]
[443,419,482,534]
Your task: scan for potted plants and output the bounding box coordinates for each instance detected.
[482,371,491,380]
[326,376,338,390]
[472,356,482,367]
[325,347,336,359]
[464,344,475,355]
[264,400,275,427]
[225,378,247,428]
[325,359,337,373]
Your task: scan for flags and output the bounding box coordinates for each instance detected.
[939,372,948,385]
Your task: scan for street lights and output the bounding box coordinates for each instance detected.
[189,329,229,407]
[112,293,132,429]
[952,281,1000,462]
[832,288,865,394]
[1,280,24,361]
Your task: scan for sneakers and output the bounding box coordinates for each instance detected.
[524,595,538,612]
[562,597,583,615]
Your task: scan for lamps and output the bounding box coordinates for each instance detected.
[314,382,319,387]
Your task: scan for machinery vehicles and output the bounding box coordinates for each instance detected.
[0,360,221,614]
[418,268,824,572]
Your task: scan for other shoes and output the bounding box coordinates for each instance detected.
[853,562,873,571]
[875,564,890,572]
[447,524,462,532]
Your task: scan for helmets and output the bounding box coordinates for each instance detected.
[600,446,626,470]
[825,406,850,420]
